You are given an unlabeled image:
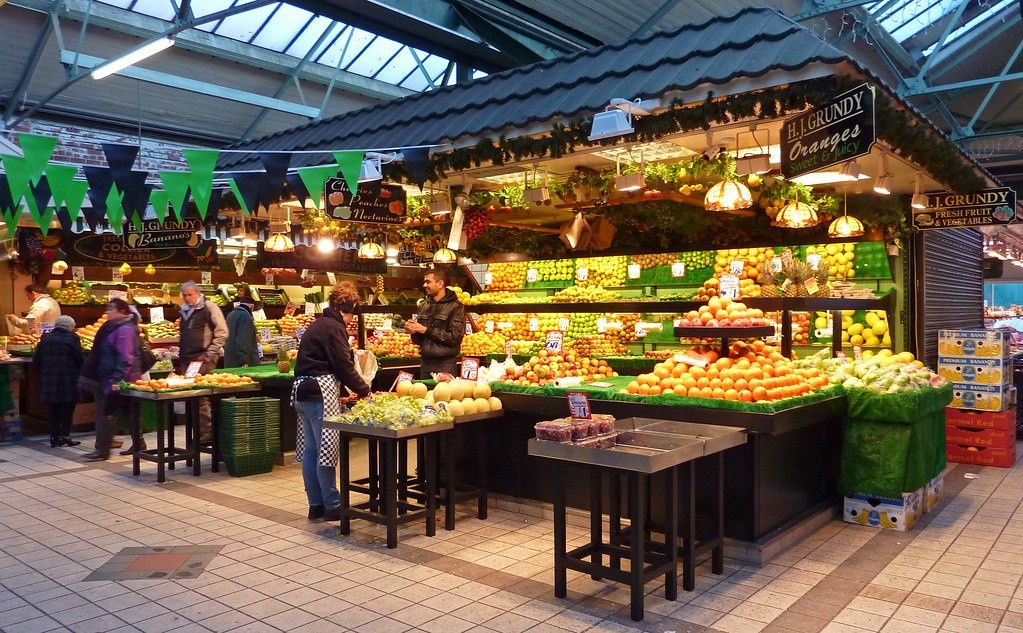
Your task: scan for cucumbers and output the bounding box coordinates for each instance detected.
[304,292,325,313]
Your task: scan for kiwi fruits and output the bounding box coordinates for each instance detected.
[611,297,652,303]
[630,253,678,270]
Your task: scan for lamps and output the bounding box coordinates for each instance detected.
[874,154,894,194]
[264,232,295,253]
[433,249,456,264]
[735,125,772,174]
[828,189,864,239]
[982,231,1023,267]
[358,242,384,259]
[775,193,818,228]
[143,204,173,220]
[704,173,752,211]
[587,97,641,141]
[616,147,646,191]
[524,162,548,202]
[911,175,930,209]
[91,33,176,80]
[358,152,397,183]
[454,183,472,206]
[838,160,861,181]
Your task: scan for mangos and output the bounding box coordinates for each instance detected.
[147,319,180,338]
[52,289,109,306]
[0,334,42,345]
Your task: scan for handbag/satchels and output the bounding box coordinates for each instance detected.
[80,333,99,383]
[31,333,46,371]
[136,333,157,375]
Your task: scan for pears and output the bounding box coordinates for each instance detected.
[713,247,776,282]
[691,278,761,301]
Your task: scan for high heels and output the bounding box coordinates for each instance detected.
[59,436,81,447]
[50,435,67,448]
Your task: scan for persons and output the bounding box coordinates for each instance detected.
[232,283,256,303]
[223,295,261,368]
[402,268,466,383]
[173,281,229,445]
[34,315,85,449]
[6,283,62,334]
[78,296,147,461]
[291,280,371,523]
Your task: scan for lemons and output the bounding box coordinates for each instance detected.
[679,183,786,215]
[575,255,628,286]
[653,292,692,302]
[553,285,618,303]
[681,251,714,271]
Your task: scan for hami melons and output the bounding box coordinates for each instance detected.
[397,380,502,418]
[844,349,925,370]
[815,311,893,347]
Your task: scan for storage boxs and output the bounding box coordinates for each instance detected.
[122,386,261,400]
[321,408,504,437]
[844,472,943,532]
[72,401,98,427]
[59,283,290,307]
[217,394,283,477]
[937,329,1017,467]
[527,416,747,473]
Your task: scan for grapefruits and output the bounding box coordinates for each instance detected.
[804,243,856,280]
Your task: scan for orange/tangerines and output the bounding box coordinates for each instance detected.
[53,262,68,270]
[118,263,155,275]
[303,217,440,247]
[678,296,772,328]
[627,341,836,404]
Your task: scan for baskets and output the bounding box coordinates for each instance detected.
[221,396,280,477]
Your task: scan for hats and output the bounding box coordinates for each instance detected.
[240,297,257,306]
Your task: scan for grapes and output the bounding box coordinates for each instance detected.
[536,414,617,451]
[338,391,455,431]
[24,234,42,255]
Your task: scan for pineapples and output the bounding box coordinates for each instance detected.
[276,345,291,373]
[759,257,831,299]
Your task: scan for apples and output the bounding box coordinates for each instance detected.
[458,291,644,386]
[129,372,252,391]
[251,313,421,357]
[484,258,573,289]
[680,312,812,345]
[77,313,109,339]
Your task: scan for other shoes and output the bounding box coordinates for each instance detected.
[119,437,146,455]
[80,449,110,462]
[95,437,123,449]
[200,433,212,441]
[323,507,358,521]
[308,504,325,520]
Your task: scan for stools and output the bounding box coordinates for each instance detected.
[554,449,725,621]
[340,420,490,549]
[131,391,263,482]
